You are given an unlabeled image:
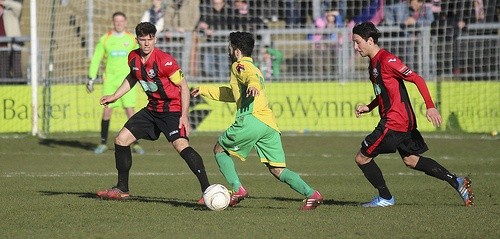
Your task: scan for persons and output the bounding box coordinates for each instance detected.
[87,12,145,154]
[193,0,500,81]
[352,21,474,207]
[0,0,24,84]
[140,0,164,43]
[97,22,210,204]
[189,32,324,211]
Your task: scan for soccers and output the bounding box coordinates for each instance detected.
[203,183,231,211]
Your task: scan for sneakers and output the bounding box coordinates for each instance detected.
[301,190,322,211]
[199,198,204,203]
[457,174,473,206]
[132,145,143,154]
[229,187,249,207]
[98,187,129,198]
[362,195,394,207]
[95,144,107,154]
